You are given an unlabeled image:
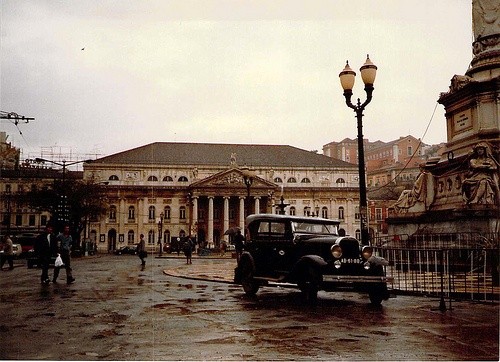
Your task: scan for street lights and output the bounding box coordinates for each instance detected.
[337,54,378,255]
[34,156,93,230]
[157,211,165,257]
[242,170,256,215]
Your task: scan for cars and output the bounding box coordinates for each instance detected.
[0,244,23,260]
[113,245,138,256]
[27,241,59,269]
[232,214,396,306]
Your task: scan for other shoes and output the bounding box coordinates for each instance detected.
[42,279,50,286]
[53,280,57,284]
[68,279,75,283]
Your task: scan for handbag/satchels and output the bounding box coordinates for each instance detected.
[140,251,147,257]
[55,254,64,267]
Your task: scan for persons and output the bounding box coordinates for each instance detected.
[0,232,13,270]
[200,241,221,253]
[52,224,75,283]
[134,234,145,266]
[233,230,244,263]
[35,224,57,285]
[388,161,426,209]
[163,243,171,254]
[223,241,228,253]
[470,142,500,204]
[337,228,346,237]
[182,235,195,264]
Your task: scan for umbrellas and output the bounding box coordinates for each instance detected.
[223,227,243,235]
